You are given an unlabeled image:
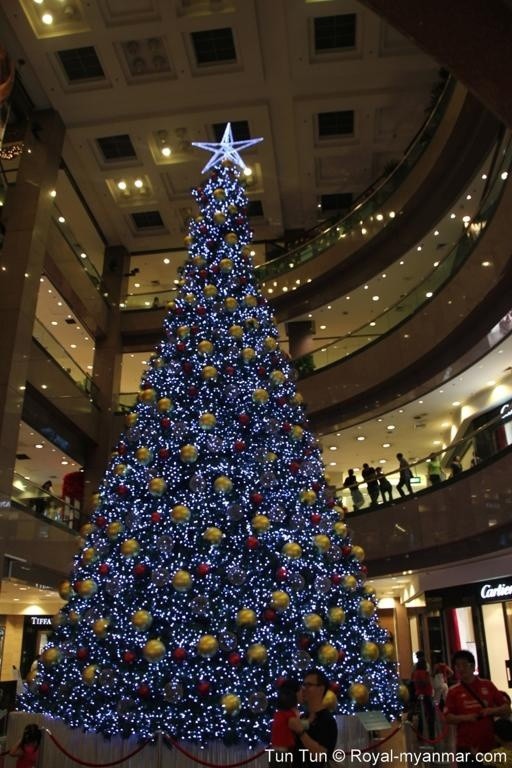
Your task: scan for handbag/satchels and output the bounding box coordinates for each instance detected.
[494,716,512,741]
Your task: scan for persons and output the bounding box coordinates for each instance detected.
[441,649,512,768]
[413,659,440,750]
[406,650,432,723]
[361,462,379,505]
[450,454,464,476]
[33,479,54,514]
[492,691,512,742]
[393,452,415,498]
[470,451,482,468]
[426,451,446,486]
[373,467,394,505]
[291,668,339,768]
[432,654,455,704]
[263,679,317,768]
[343,468,365,512]
[469,716,512,768]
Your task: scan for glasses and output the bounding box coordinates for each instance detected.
[301,682,320,690]
[453,662,474,671]
